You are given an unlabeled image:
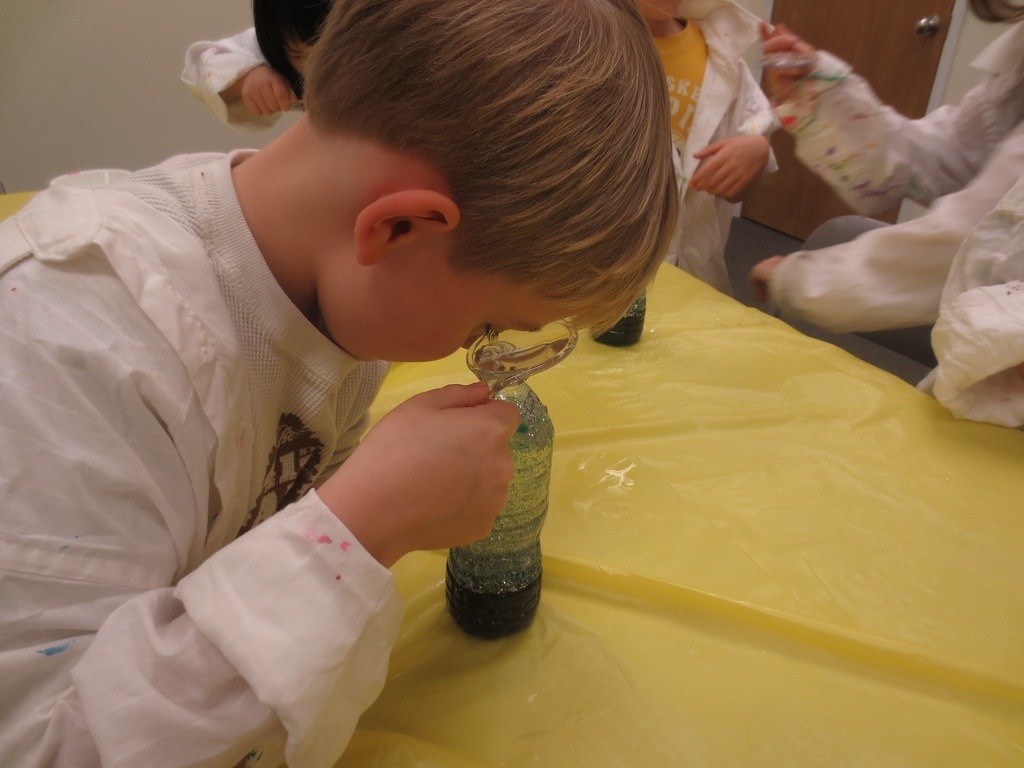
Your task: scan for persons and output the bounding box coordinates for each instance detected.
[0,1,684,768]
[183,0,1024,427]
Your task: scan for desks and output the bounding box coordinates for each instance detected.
[0,192,1024,768]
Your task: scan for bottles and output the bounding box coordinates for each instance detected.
[444,340,554,641]
[589,284,646,347]
